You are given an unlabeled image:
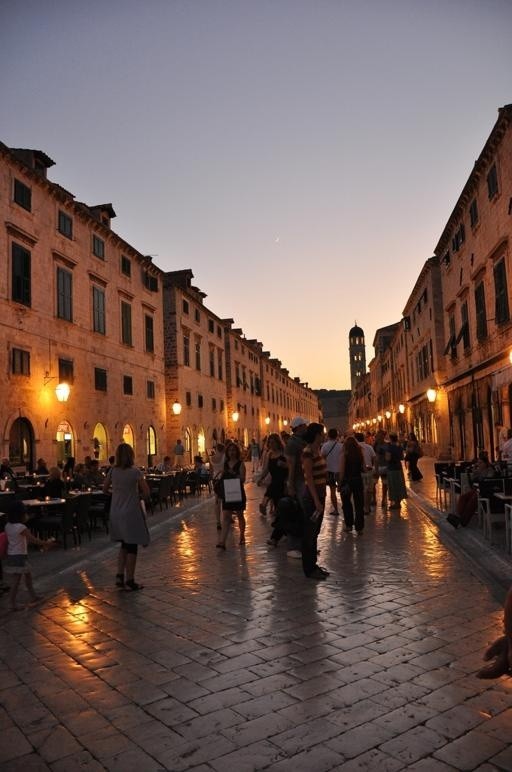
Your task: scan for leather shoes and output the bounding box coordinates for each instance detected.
[343,526,364,536]
[305,566,329,580]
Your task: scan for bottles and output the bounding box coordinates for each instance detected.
[310,505,323,523]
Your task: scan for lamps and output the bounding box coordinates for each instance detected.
[399,400,406,414]
[43,375,71,403]
[426,384,438,403]
[171,397,182,417]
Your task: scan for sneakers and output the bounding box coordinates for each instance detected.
[267,539,278,548]
[330,510,340,516]
[287,550,303,559]
[239,538,245,545]
[216,544,226,551]
[364,500,402,515]
[31,593,44,601]
[446,513,467,530]
[259,503,277,515]
[231,519,235,523]
[8,602,26,611]
[216,524,222,530]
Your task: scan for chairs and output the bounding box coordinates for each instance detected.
[433,458,511,552]
[0,462,210,554]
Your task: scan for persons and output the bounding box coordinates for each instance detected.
[102,442,152,592]
[475,586,512,681]
[212,439,246,550]
[248,417,423,579]
[476,422,511,477]
[4,500,57,612]
[0,439,212,499]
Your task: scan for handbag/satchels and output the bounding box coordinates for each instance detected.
[277,459,288,469]
[213,471,223,486]
[418,447,423,458]
[362,464,372,473]
[0,531,8,561]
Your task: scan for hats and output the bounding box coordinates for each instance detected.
[291,416,310,430]
[344,429,355,438]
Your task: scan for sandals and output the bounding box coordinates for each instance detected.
[125,580,144,591]
[115,574,126,587]
